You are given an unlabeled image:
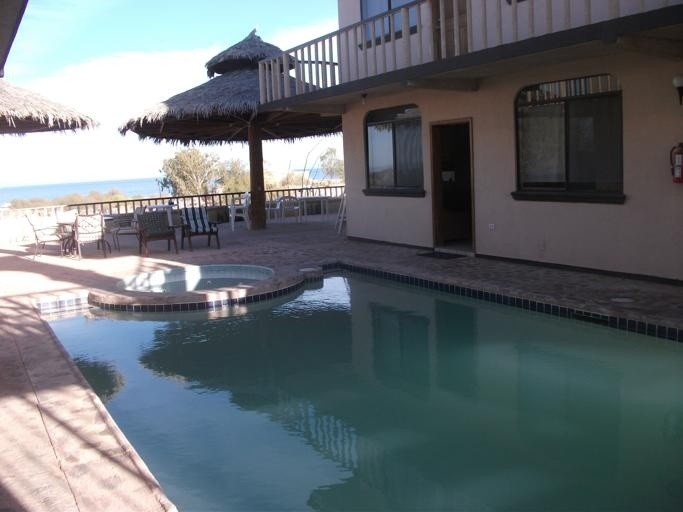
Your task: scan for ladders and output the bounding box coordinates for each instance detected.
[334,186,346,233]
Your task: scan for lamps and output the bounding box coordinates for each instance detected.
[672,75,683,104]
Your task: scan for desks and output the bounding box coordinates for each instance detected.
[298,196,329,222]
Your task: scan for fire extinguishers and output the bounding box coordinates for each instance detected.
[669,142,683,183]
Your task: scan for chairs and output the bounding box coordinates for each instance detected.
[23,191,299,258]
